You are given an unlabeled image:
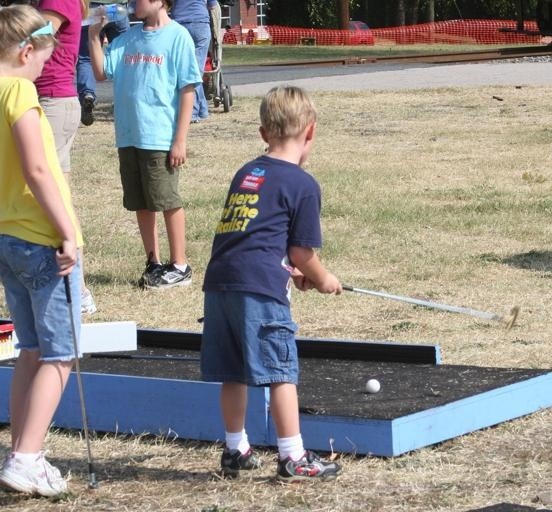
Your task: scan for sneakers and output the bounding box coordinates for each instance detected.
[221,446,267,475]
[276,450,340,484]
[0,451,69,496]
[80,92,95,126]
[137,252,192,289]
[80,290,97,315]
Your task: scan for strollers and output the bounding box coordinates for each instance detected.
[201,1,235,111]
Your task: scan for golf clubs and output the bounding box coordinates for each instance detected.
[342,284,518,330]
[57,245,97,492]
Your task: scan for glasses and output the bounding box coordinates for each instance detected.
[19,20,54,47]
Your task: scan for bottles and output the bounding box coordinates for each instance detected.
[80,3,135,28]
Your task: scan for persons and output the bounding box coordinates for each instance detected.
[167,0,221,123]
[77,1,131,125]
[88,1,203,290]
[34,1,97,315]
[0,4,84,500]
[197,83,343,482]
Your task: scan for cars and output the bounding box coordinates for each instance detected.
[315,20,374,47]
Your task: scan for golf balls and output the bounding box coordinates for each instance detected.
[366,379,381,393]
